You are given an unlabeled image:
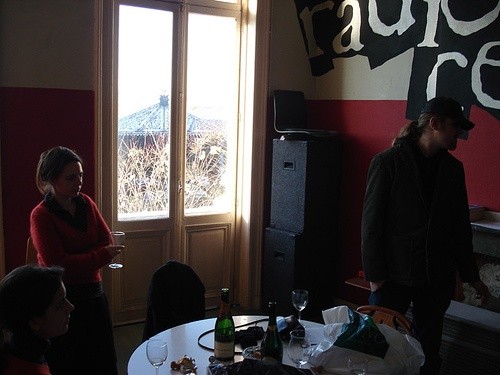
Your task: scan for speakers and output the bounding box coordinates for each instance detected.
[264,139,344,324]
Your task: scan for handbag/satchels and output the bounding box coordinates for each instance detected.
[287,305,425,375]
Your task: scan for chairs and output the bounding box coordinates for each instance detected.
[356,305,409,333]
[143,260,206,342]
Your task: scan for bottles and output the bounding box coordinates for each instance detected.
[260,300,282,363]
[214,288,235,364]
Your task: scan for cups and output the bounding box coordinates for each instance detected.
[287,329,311,369]
[348,358,369,375]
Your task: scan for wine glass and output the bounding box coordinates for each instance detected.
[291,289,308,329]
[108,231,126,269]
[146,339,168,375]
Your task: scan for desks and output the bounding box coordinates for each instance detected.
[127,316,412,375]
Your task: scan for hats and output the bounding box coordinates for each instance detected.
[422,95,475,132]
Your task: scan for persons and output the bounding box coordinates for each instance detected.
[361,96,488,374]
[0,263,76,375]
[29,146,125,375]
